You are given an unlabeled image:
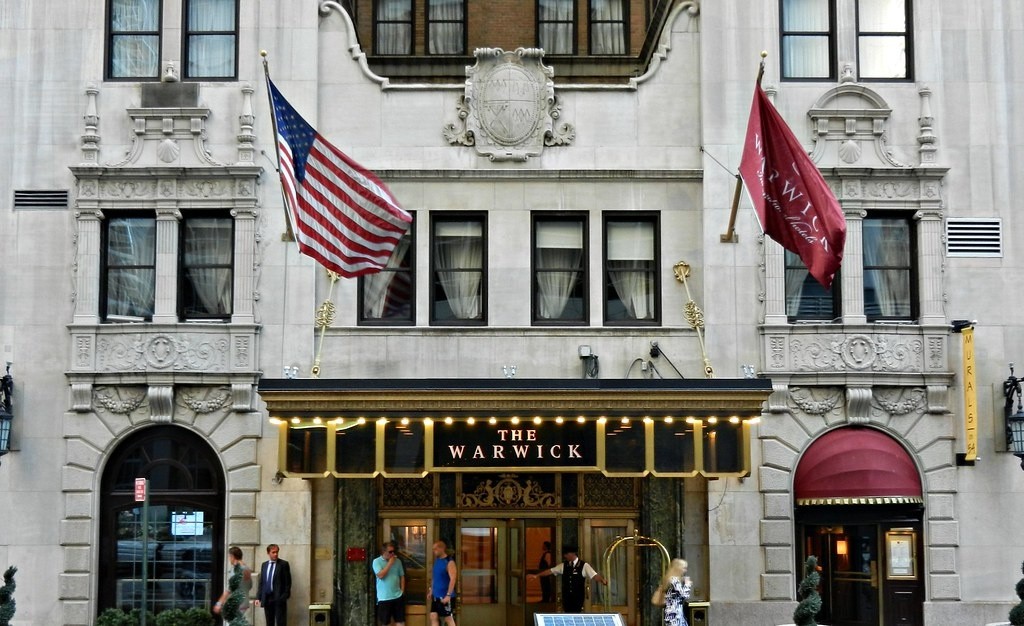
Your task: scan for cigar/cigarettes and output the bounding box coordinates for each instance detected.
[394,556,397,557]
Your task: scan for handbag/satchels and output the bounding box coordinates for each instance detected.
[651,585,667,608]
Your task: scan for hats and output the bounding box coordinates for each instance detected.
[563,545,578,553]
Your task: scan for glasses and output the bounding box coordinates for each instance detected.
[384,550,396,554]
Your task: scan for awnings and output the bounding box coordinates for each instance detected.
[795,428,922,505]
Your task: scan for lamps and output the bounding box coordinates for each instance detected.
[1003,363,1024,470]
[578,345,591,360]
[0,365,14,465]
[837,540,848,554]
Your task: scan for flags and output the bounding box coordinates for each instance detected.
[739,80,847,290]
[266,75,412,278]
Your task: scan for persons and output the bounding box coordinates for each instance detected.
[426,541,458,626]
[538,541,552,603]
[255,544,292,626]
[213,547,252,615]
[372,541,406,626]
[663,558,692,626]
[530,545,608,612]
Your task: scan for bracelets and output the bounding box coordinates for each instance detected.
[447,593,451,596]
[216,602,222,606]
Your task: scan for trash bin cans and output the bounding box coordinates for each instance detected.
[309,605,331,626]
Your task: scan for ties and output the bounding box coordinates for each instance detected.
[571,561,573,570]
[266,562,275,595]
